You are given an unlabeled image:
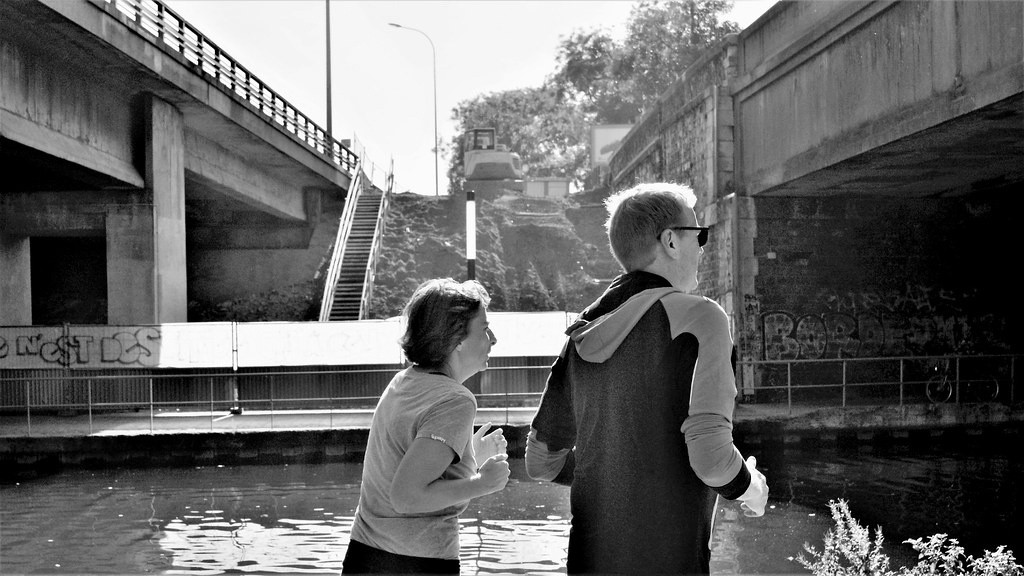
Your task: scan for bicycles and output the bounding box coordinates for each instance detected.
[925,353,999,404]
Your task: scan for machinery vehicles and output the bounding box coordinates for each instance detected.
[461,127,527,201]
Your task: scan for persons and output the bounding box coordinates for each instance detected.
[341,278,511,576]
[946,320,988,390]
[524,178,769,576]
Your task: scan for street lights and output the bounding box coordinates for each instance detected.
[389,23,438,196]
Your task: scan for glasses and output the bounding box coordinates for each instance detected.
[657,225,710,247]
